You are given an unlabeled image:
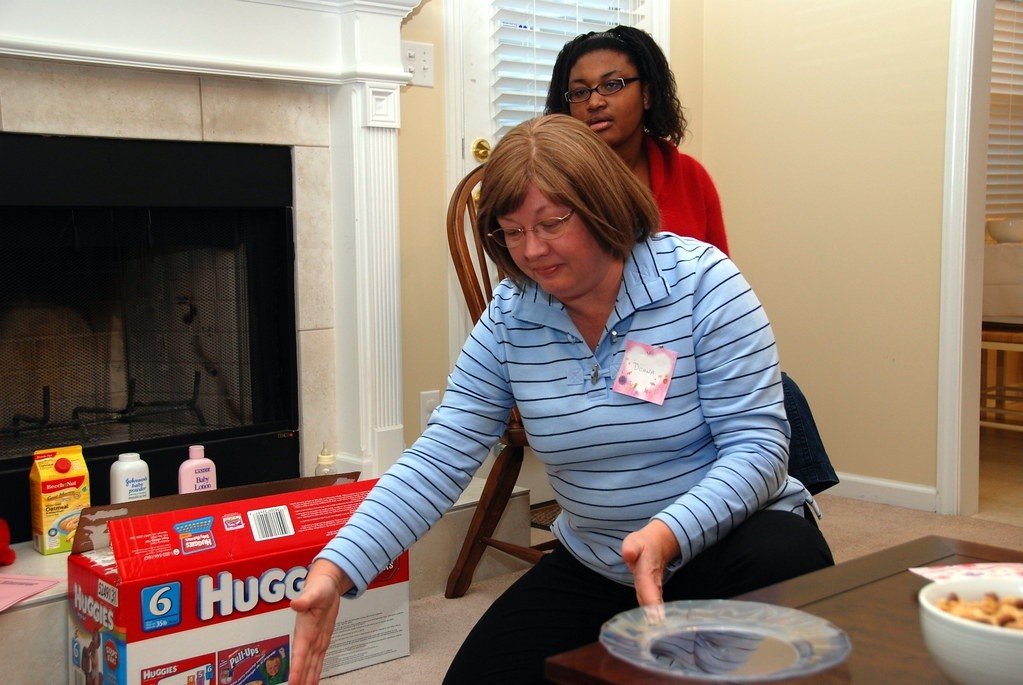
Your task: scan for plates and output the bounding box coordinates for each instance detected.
[598,599,853,683]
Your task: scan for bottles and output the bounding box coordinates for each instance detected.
[315,441,337,475]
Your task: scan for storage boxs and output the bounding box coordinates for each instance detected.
[68,470,409,685]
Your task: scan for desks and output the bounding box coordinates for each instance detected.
[540,534,1023,685]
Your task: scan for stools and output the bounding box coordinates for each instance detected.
[982,315,1023,431]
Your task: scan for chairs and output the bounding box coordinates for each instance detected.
[444,158,558,598]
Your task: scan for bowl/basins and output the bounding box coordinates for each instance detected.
[918,575,1023,685]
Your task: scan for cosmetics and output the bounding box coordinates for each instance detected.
[110,452,151,503]
[179,445,217,494]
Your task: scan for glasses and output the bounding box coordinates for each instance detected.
[565,77,638,103]
[487,209,574,248]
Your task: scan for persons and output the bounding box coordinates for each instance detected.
[542,27,841,501]
[287,114,834,685]
